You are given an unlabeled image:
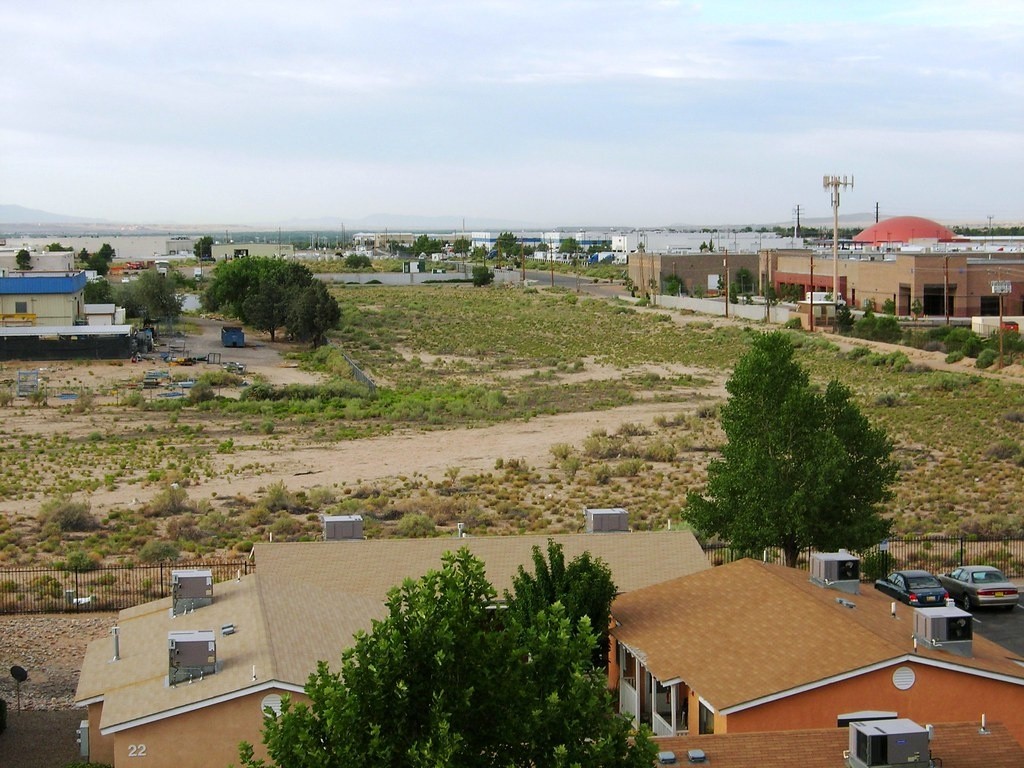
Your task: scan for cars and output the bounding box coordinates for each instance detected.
[875,571,949,607]
[938,565,1020,611]
